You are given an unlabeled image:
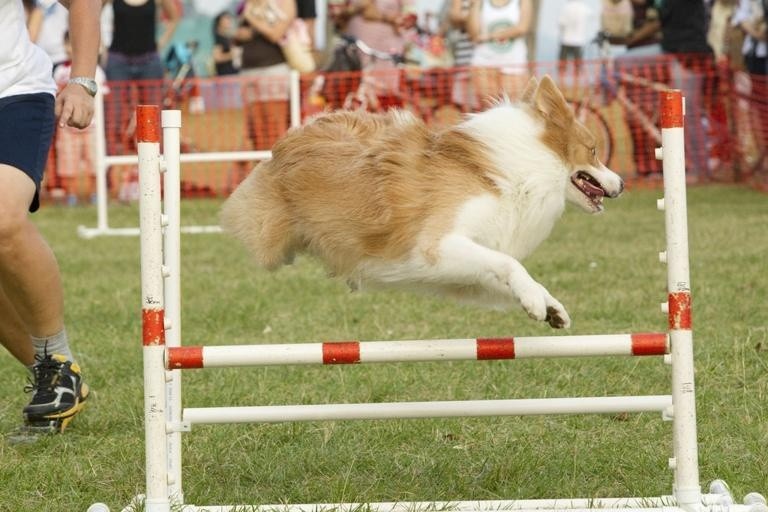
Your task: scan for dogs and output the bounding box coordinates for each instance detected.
[215,73,624,330]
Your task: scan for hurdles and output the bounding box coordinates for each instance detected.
[77,72,302,240]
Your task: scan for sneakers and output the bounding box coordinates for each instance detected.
[18,354,94,435]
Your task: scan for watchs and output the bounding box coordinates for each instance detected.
[69,75,97,98]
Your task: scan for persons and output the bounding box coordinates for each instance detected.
[2,0,100,438]
[23,0,60,50]
[47,32,110,205]
[95,2,178,167]
